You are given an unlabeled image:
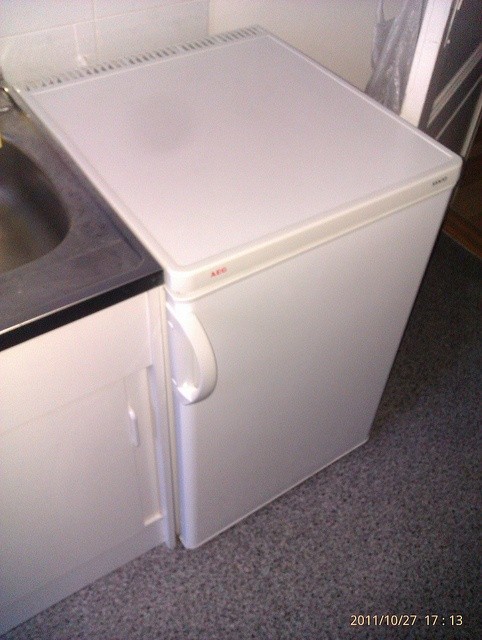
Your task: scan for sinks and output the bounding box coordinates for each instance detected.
[1,72,72,289]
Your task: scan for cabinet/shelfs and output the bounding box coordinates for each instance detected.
[1,268,177,636]
